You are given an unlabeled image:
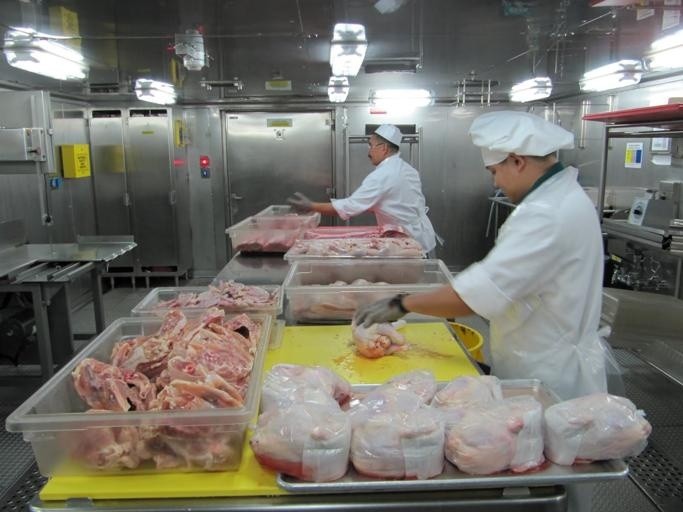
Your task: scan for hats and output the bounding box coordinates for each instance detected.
[374,124,403,146]
[468,110,575,168]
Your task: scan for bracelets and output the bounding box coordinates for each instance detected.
[389,292,410,316]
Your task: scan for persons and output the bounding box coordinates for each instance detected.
[284,122,440,262]
[353,110,609,512]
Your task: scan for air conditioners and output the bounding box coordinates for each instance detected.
[131,77,178,108]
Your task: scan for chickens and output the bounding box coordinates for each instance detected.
[350,318,407,359]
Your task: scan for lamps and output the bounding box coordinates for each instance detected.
[507,75,553,104]
[329,22,370,79]
[577,60,642,94]
[325,75,351,104]
[642,44,683,74]
[0,46,88,84]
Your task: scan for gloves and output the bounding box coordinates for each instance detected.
[286,193,312,210]
[352,293,407,328]
